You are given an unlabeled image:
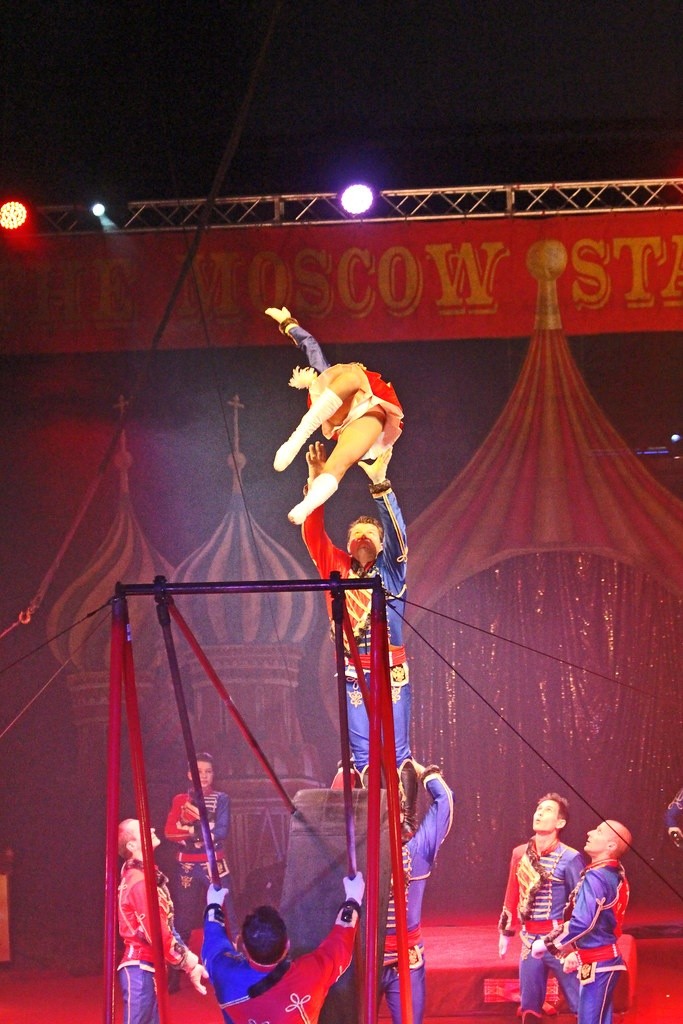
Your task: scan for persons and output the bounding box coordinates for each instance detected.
[664,788,683,848]
[330,757,453,1024]
[265,307,404,523]
[201,872,365,1024]
[498,794,632,1024]
[117,753,239,1024]
[301,442,417,844]
